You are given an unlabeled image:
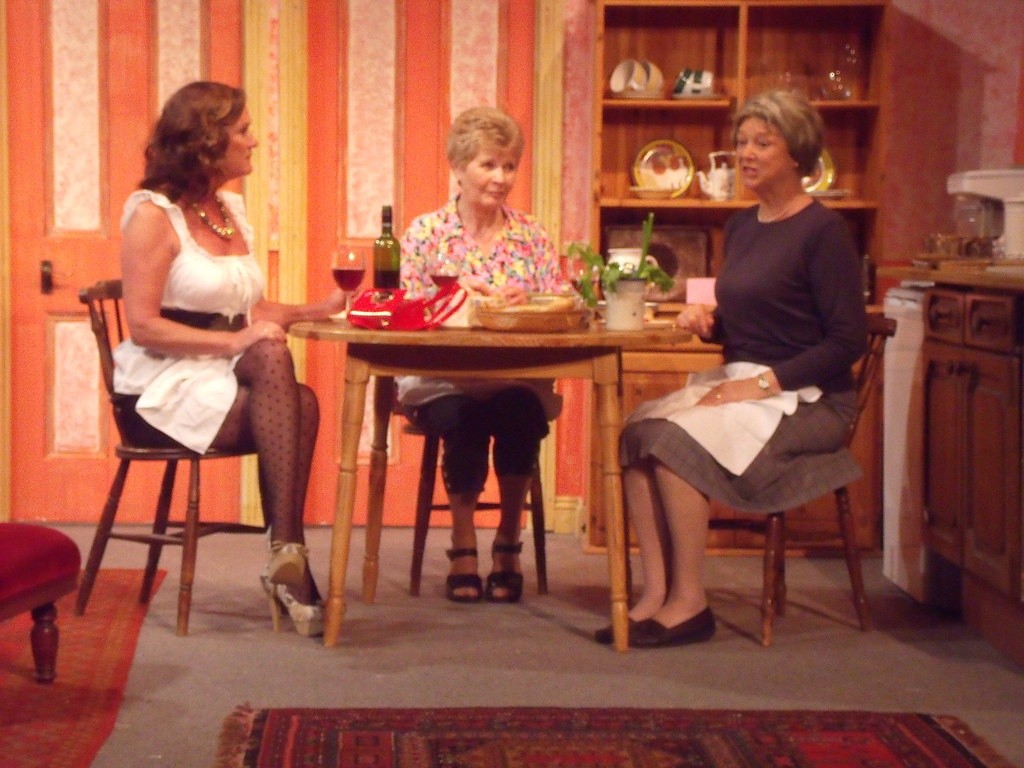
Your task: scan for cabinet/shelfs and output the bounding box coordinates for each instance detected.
[576,1,882,555]
[926,272,1024,675]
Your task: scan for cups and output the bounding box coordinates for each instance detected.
[748,66,853,101]
[603,277,647,331]
[429,251,461,291]
[609,58,714,96]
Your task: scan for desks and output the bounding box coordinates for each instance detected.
[290,314,691,650]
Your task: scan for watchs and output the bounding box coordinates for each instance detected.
[757,374,773,398]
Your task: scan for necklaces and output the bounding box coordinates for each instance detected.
[184,195,236,239]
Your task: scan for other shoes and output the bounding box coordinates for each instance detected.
[626,607,714,648]
[594,615,633,643]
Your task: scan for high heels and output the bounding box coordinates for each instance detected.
[265,540,310,598]
[446,547,481,601]
[484,540,524,603]
[260,575,327,638]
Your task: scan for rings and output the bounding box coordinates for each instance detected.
[715,392,722,400]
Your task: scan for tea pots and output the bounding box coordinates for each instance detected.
[695,149,738,201]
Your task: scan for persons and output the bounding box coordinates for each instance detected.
[113,82,326,636]
[594,92,868,649]
[390,106,590,605]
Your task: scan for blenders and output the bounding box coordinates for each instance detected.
[933,165,1024,263]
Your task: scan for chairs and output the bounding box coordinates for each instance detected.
[399,422,551,597]
[79,280,295,638]
[0,524,83,682]
[707,311,901,646]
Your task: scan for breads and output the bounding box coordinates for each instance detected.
[502,297,575,311]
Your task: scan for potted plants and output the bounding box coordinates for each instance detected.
[567,208,672,332]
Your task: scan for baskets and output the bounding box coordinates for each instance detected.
[477,304,592,332]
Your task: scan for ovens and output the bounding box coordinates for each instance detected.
[882,289,930,605]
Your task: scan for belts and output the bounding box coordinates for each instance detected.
[161,308,249,334]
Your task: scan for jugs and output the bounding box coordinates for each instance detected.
[605,248,659,290]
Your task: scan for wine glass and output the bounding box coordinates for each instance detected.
[330,244,366,315]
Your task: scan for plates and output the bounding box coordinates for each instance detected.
[634,138,694,198]
[801,147,839,192]
[808,189,852,202]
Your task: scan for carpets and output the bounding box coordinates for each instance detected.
[215,706,1020,768]
[0,570,167,768]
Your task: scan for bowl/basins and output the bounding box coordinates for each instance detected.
[630,186,678,199]
[472,307,586,333]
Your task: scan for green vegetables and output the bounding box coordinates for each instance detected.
[564,213,674,309]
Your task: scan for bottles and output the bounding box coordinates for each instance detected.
[372,204,400,288]
